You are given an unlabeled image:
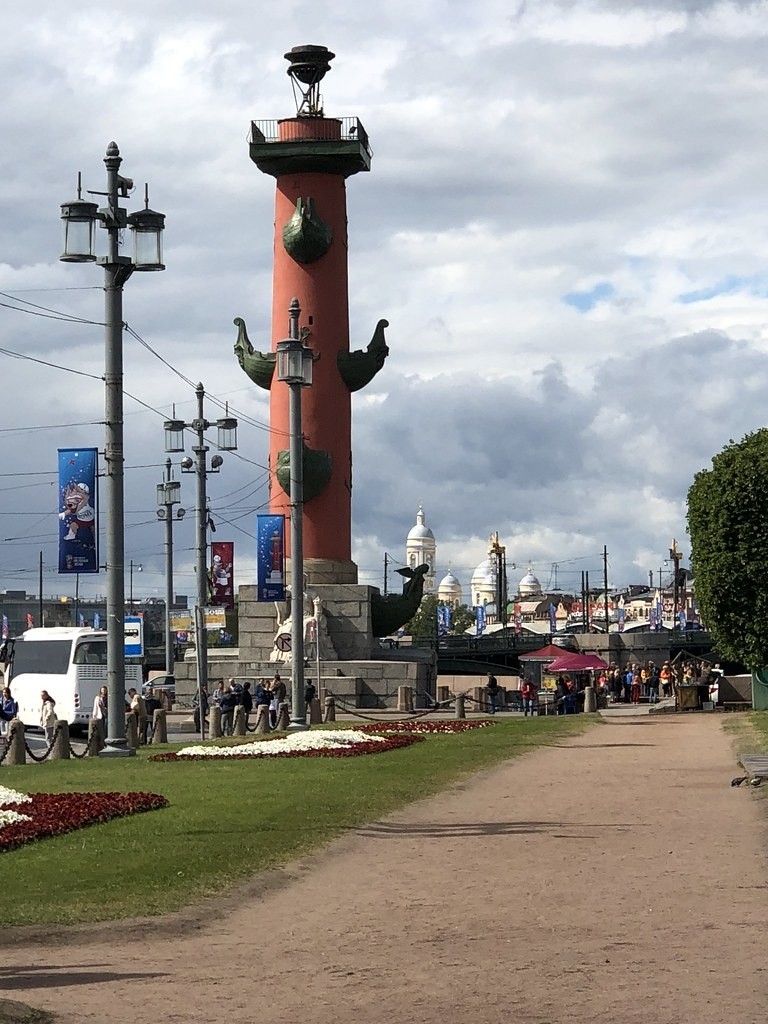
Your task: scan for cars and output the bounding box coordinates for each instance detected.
[552,634,578,647]
[380,638,400,650]
[142,675,175,698]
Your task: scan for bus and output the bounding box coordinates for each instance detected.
[0,626,142,734]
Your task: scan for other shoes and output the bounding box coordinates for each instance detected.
[649,702,656,704]
[196,729,200,732]
[490,711,495,714]
[633,701,640,704]
[616,702,620,704]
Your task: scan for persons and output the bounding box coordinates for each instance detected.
[0,687,15,735]
[192,674,316,737]
[555,673,577,714]
[92,686,162,745]
[487,672,498,714]
[521,676,536,717]
[40,690,56,749]
[599,661,724,705]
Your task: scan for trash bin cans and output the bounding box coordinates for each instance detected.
[675,684,710,711]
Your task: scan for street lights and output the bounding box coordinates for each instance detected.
[162,380,238,706]
[276,294,317,729]
[60,143,166,760]
[156,456,186,674]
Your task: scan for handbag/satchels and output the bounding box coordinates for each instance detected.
[530,690,537,700]
[487,688,498,696]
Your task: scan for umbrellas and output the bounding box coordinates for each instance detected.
[518,644,609,714]
